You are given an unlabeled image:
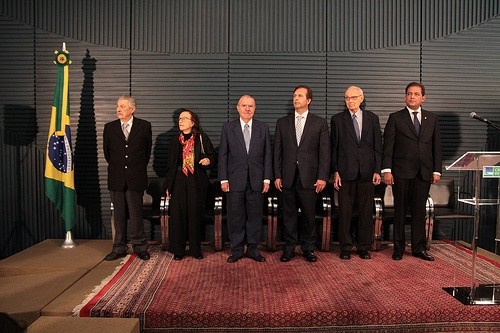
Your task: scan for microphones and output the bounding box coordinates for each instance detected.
[470,112,498,129]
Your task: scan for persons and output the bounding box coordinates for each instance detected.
[102,96,153,261]
[380,82,441,261]
[270,86,330,262]
[152,110,218,260]
[217,94,272,263]
[329,86,383,260]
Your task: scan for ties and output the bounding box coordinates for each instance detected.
[296,117,302,144]
[123,122,129,140]
[411,112,421,131]
[243,124,251,153]
[353,114,361,141]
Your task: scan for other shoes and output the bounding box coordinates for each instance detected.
[137,251,150,260]
[392,249,403,260]
[189,250,203,259]
[357,249,370,259]
[340,250,350,259]
[412,251,434,260]
[280,252,295,262]
[228,255,243,262]
[174,253,185,260]
[106,252,127,261]
[303,252,317,262]
[245,253,266,261]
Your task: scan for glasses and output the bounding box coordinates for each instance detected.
[345,95,362,101]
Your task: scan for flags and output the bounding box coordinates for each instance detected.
[44,49,74,231]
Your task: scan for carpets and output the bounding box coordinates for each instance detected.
[71,238,500,333]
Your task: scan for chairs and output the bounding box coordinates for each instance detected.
[108,177,478,252]
